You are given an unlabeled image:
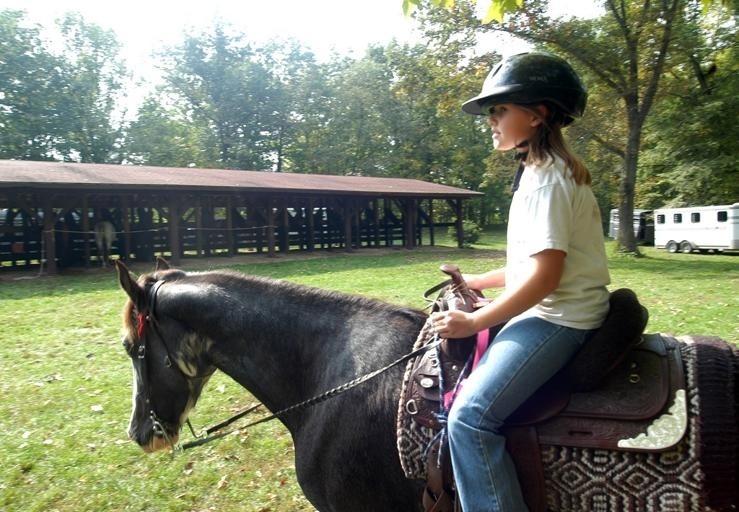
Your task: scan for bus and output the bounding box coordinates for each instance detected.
[653,202,738,254]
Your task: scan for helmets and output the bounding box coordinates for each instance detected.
[461,50,589,118]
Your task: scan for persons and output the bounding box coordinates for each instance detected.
[429,52,612,511]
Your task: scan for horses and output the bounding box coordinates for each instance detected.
[116,256,739,512]
[92,219,117,270]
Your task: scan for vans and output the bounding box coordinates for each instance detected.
[608,207,654,244]
